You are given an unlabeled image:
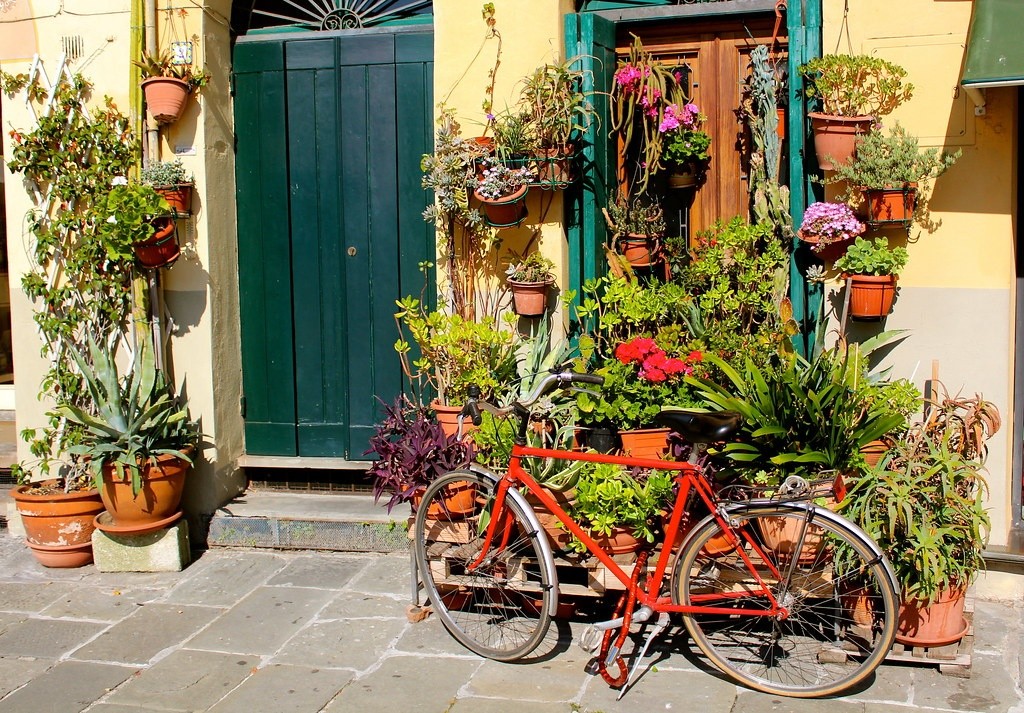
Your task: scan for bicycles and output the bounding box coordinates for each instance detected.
[415,362,903,703]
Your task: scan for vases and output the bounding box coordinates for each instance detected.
[666,155,696,189]
[617,428,673,461]
[473,173,526,230]
[796,223,867,262]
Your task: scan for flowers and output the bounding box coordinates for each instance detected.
[616,333,710,432]
[616,63,713,169]
[476,156,533,199]
[461,98,536,168]
[799,203,862,240]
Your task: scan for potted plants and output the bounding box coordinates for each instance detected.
[363,53,1003,647]
[0,49,211,569]
